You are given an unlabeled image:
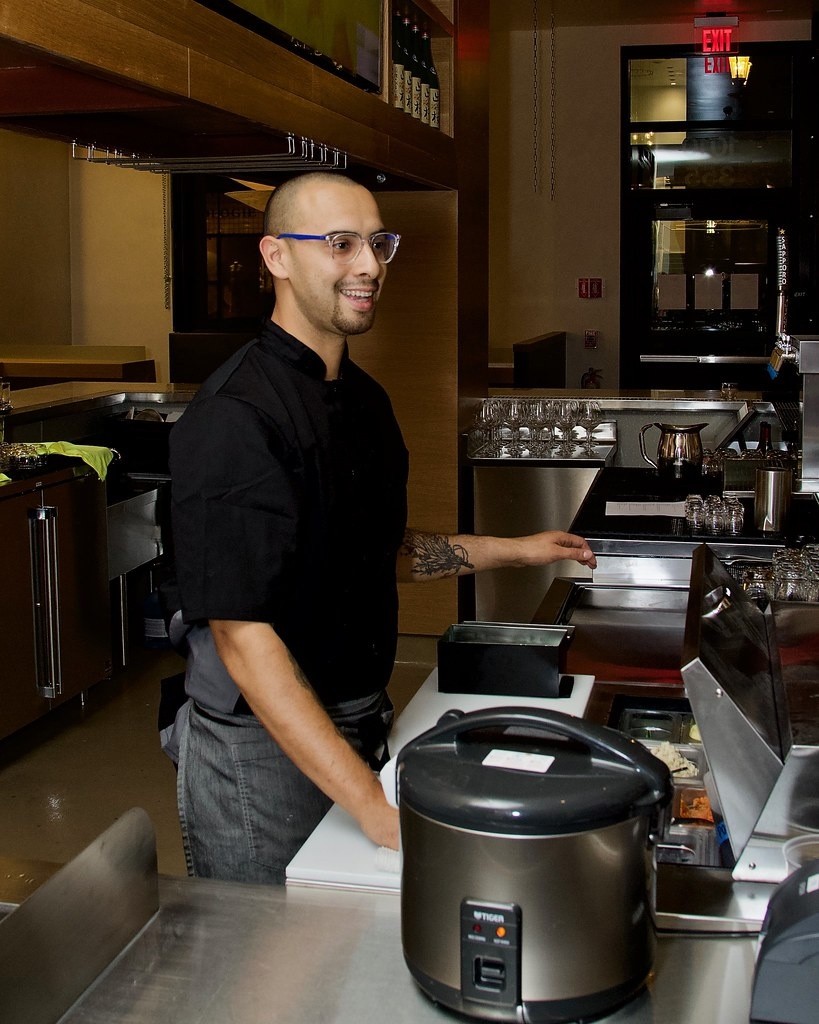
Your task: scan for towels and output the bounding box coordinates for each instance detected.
[3,440,114,483]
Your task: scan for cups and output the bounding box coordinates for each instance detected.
[703,447,797,475]
[719,381,737,401]
[0,441,48,470]
[682,492,744,535]
[1,383,14,411]
[743,544,819,602]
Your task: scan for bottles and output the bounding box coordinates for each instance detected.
[759,420,774,450]
[387,4,443,128]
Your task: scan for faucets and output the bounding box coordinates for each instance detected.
[767,333,795,380]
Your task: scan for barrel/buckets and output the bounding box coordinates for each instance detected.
[755,465,792,532]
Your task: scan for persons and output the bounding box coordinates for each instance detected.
[169,172,597,885]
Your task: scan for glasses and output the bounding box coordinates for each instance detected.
[278,231,402,265]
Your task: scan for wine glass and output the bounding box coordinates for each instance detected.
[476,396,600,459]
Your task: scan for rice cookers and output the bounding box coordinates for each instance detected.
[378,707,678,1024]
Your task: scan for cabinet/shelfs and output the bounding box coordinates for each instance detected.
[470,464,601,624]
[83,0,458,165]
[0,472,116,740]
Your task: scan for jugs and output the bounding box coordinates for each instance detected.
[636,421,710,478]
